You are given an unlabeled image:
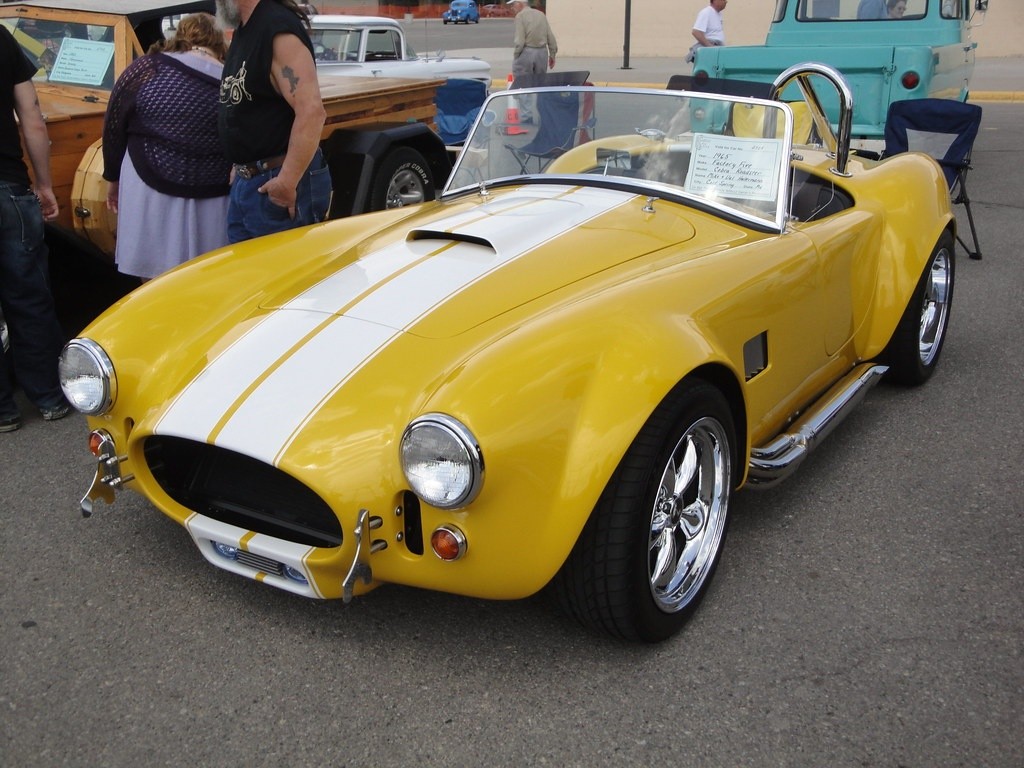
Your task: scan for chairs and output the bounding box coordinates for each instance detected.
[879,98,983,260]
[787,181,852,224]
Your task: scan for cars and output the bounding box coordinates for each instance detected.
[300,13,494,99]
[442,0,481,25]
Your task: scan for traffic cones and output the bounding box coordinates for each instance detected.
[497,72,530,135]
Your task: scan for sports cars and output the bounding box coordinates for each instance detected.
[55,59,957,643]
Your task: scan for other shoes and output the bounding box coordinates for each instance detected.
[1,418,25,435]
[41,407,70,421]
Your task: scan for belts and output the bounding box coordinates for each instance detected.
[233,154,289,180]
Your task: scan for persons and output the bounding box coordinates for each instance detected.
[102,12,232,284]
[0,25,70,433]
[685,0,727,63]
[857,0,907,19]
[506,0,558,123]
[216,0,332,244]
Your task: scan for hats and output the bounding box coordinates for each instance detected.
[506,0,528,4]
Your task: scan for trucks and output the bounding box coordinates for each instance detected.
[686,0,991,142]
[0,0,454,272]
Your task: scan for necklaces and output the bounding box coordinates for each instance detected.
[191,47,213,56]
